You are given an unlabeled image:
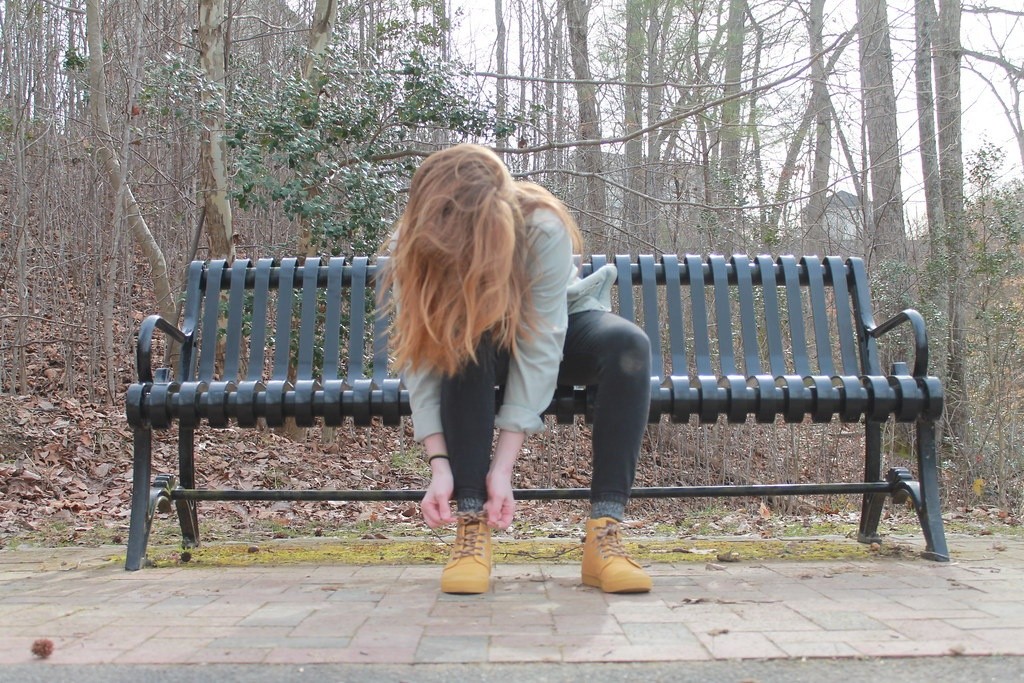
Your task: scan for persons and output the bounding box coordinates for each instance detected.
[370,143,652,594]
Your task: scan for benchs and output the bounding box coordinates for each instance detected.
[124,253,952,562]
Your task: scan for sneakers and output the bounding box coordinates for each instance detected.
[581,517,653,594]
[440,510,492,594]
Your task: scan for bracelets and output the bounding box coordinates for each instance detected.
[429,455,449,464]
